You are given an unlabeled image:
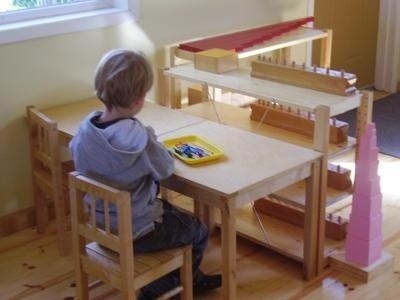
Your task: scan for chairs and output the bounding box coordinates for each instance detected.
[26,105,194,300]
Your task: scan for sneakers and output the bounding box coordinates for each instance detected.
[190,274,221,294]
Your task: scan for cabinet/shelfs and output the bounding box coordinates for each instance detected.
[161,63,374,242]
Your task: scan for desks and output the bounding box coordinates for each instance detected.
[39,99,324,300]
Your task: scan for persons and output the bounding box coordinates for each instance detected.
[67,47,221,299]
